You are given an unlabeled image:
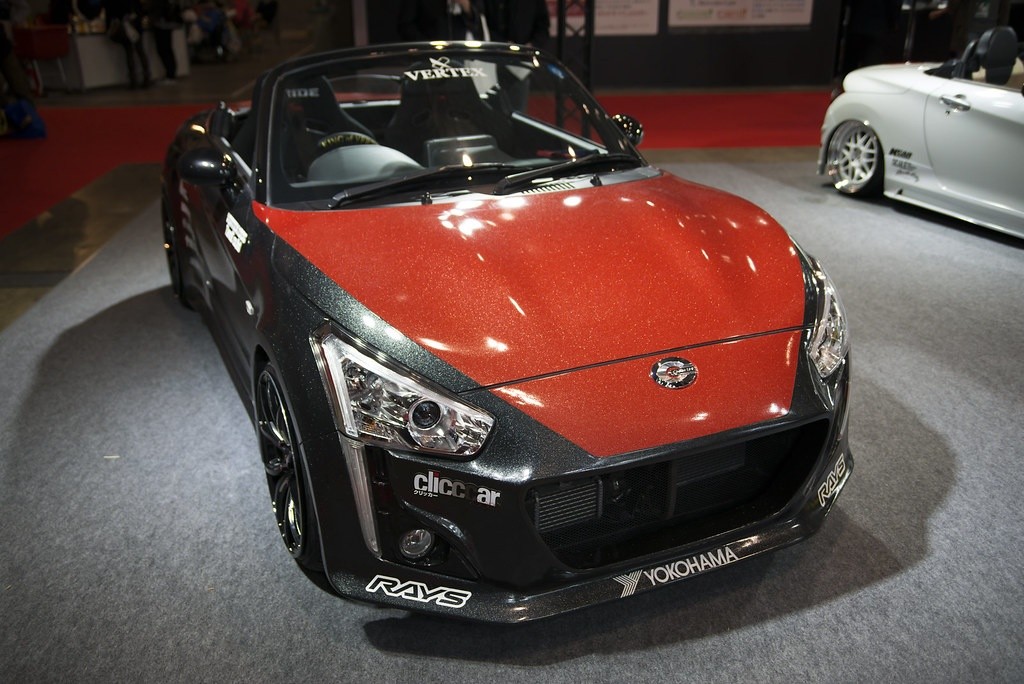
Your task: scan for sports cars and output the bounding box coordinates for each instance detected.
[816,23,1024,241]
[160,41,855,631]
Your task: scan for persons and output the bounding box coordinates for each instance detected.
[181,0,282,66]
[485,1,550,115]
[395,0,486,41]
[905,0,951,60]
[149,0,184,87]
[103,0,153,88]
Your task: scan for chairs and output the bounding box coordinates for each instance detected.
[967,25,1018,84]
[234,70,374,177]
[385,60,518,167]
